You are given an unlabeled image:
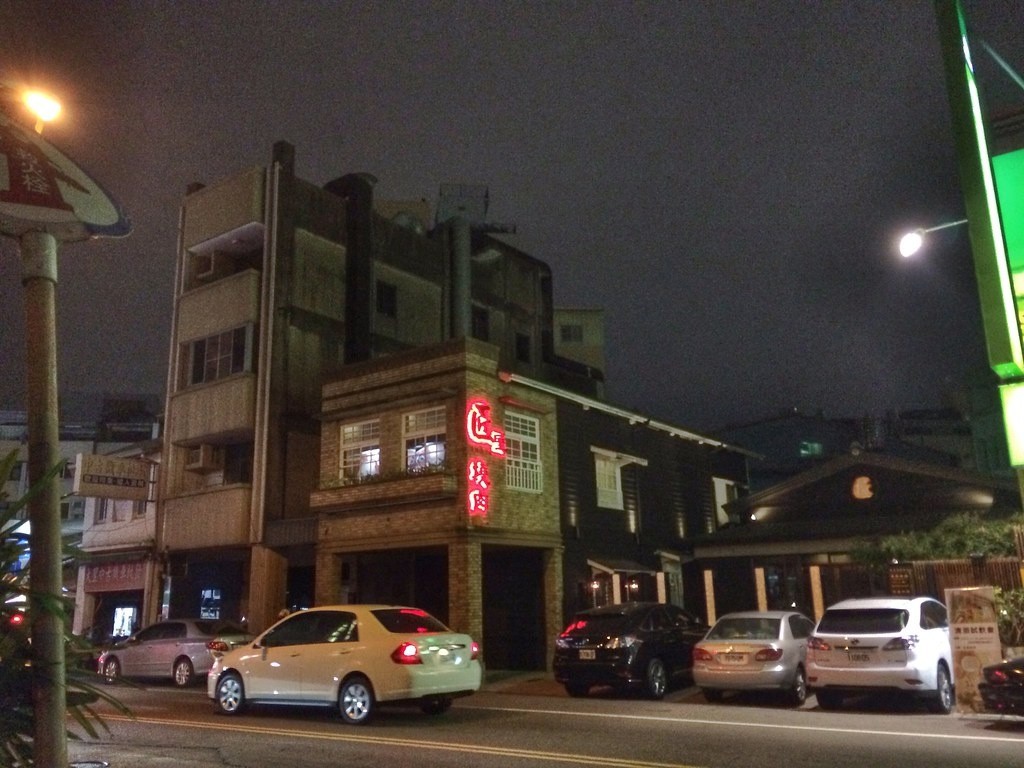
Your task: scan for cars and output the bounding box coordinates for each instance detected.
[94,615,262,688]
[692,610,817,706]
[979,658,1024,717]
[203,600,482,727]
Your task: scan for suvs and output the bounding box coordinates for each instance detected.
[551,601,714,701]
[802,595,955,714]
